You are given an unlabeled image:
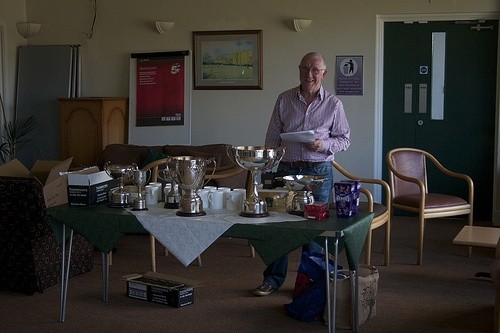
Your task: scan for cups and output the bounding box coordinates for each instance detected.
[197,186,247,211]
[144,181,182,205]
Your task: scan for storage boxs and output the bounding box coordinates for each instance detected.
[121,270,202,308]
[258,190,294,211]
[333,180,361,218]
[0,157,117,207]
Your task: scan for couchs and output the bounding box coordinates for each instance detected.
[0,178,93,292]
[99,143,253,190]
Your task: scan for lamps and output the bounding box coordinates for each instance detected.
[153,21,175,35]
[291,19,313,32]
[16,23,42,38]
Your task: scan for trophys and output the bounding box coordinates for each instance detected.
[273,174,330,216]
[157,155,217,217]
[225,144,286,218]
[104,161,151,211]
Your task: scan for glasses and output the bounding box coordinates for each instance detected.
[298,65,326,74]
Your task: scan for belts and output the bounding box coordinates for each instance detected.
[279,161,332,168]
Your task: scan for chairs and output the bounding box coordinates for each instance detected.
[381,148,474,266]
[332,161,391,267]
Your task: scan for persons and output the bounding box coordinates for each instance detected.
[255,51,351,295]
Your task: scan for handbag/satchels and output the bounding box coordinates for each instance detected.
[284,251,345,322]
[324,266,380,329]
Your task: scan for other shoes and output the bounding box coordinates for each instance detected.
[253,281,274,295]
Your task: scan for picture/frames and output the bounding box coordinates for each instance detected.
[192,29,264,91]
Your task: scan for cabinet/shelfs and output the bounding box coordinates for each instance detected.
[55,97,129,165]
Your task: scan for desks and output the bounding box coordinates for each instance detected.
[46,202,376,333]
[453,225,500,248]
